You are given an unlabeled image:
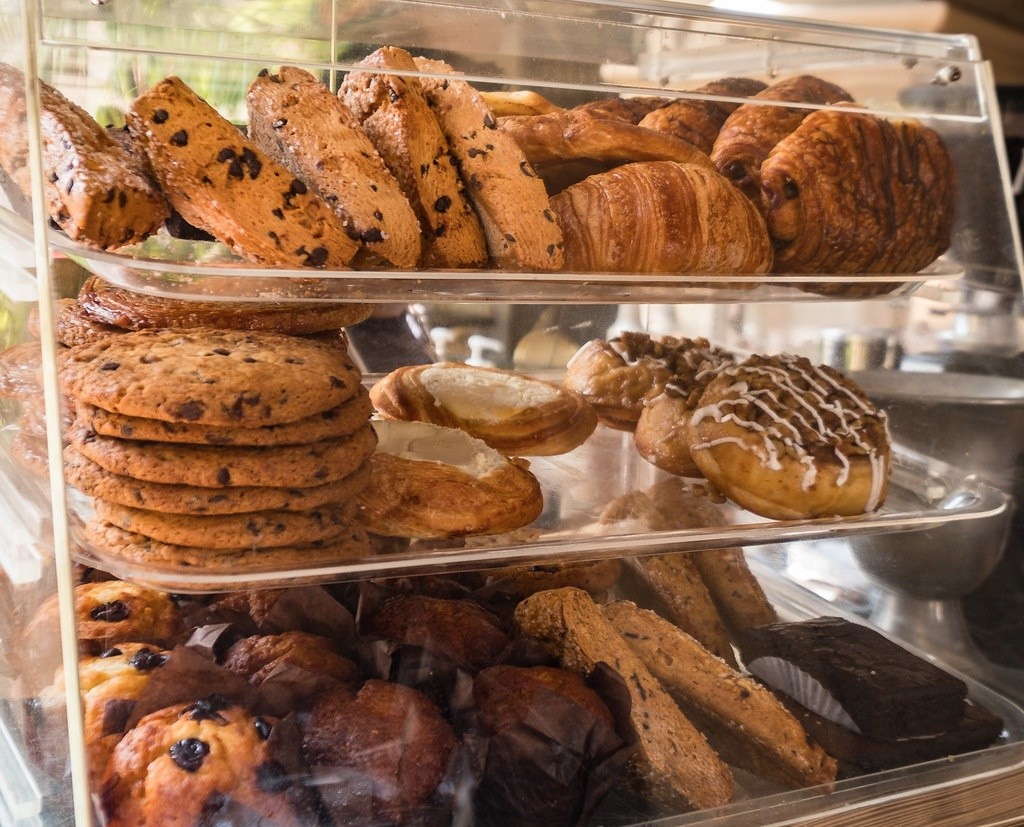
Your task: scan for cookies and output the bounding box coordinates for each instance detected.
[0,269,378,573]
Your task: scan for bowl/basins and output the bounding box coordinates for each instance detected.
[848,505,1015,603]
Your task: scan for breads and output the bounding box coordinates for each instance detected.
[0,477,970,827]
[0,42,959,301]
[366,330,891,539]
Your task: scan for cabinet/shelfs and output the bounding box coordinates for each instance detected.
[0,0,1024,827]
